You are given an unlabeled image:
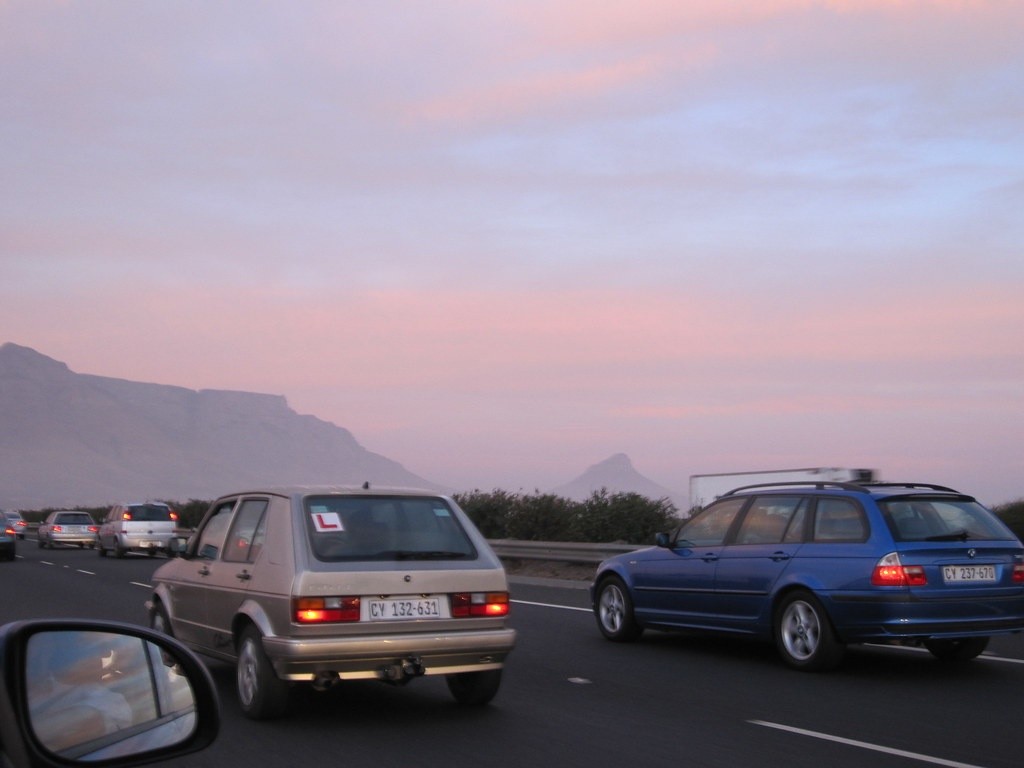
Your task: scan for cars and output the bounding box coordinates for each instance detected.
[3,510,27,541]
[0,511,17,560]
[95,503,181,560]
[37,512,99,550]
[148,488,516,709]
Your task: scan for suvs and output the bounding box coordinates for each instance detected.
[591,481,1024,670]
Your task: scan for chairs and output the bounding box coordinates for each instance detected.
[899,517,930,540]
[747,514,788,544]
